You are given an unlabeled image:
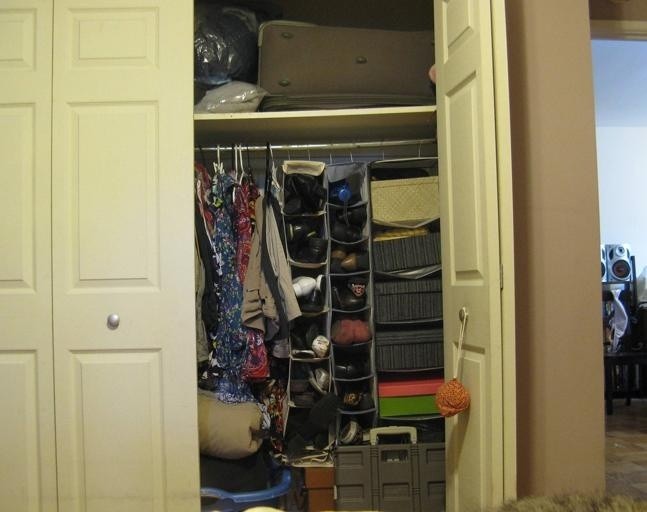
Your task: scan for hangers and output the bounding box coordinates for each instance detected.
[197,135,440,208]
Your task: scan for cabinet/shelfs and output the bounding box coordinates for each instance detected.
[1,1,201,512]
[193,1,440,116]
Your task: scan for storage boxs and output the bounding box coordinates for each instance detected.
[376,328,444,373]
[376,379,445,418]
[372,279,443,325]
[304,464,333,510]
[372,232,442,274]
[332,442,446,511]
[370,176,441,228]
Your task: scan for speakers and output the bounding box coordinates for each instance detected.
[600,244,607,282]
[605,244,633,282]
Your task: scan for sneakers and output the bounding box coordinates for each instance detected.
[284,208,379,446]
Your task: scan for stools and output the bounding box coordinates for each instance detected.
[605,352,647,415]
